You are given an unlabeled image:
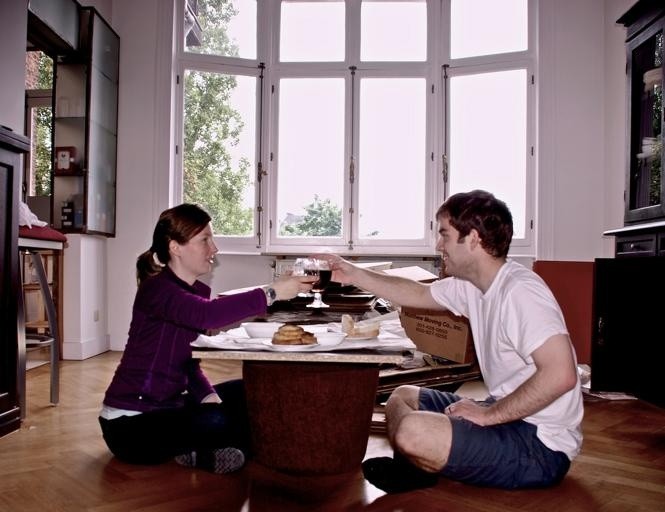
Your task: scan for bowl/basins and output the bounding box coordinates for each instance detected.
[240,322,286,338]
[641,137,658,152]
[314,331,347,346]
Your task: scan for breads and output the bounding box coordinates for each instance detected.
[272,325,317,345]
[341,313,380,338]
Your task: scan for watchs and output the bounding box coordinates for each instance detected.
[263,287,276,306]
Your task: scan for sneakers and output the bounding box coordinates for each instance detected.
[175,448,245,473]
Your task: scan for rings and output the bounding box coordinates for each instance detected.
[449,407,452,413]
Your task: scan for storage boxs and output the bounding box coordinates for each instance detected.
[381,265,477,364]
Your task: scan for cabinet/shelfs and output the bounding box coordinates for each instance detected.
[532,1,664,411]
[50,5,121,238]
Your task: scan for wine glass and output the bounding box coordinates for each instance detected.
[295,257,332,309]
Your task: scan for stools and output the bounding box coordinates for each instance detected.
[19,220,66,405]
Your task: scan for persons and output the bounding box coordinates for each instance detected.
[308,189,584,494]
[99,204,320,474]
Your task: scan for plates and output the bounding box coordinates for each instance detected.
[344,336,377,341]
[637,151,659,159]
[642,68,665,86]
[266,338,320,350]
